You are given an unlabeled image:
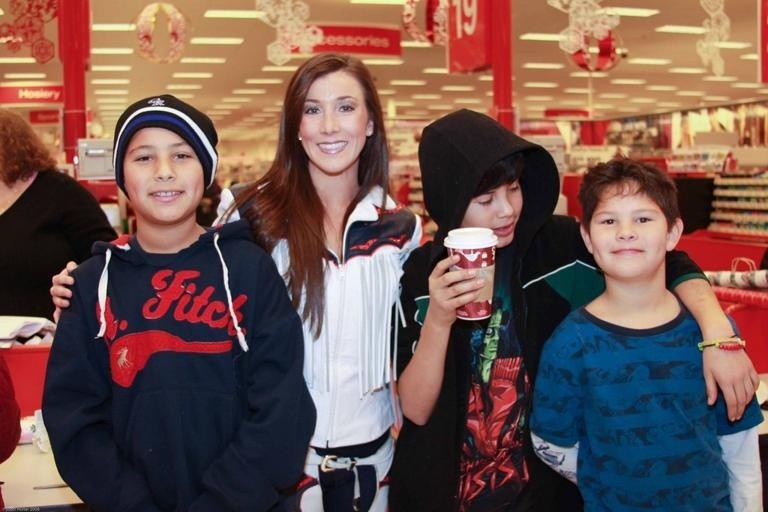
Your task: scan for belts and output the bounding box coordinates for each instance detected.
[305,437,395,472]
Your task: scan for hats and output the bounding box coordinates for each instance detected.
[112,94,218,203]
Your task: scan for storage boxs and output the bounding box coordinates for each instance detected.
[0,341,51,418]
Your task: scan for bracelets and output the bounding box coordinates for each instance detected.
[698,334,746,352]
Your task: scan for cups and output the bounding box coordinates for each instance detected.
[443,226,500,320]
[31,408,52,454]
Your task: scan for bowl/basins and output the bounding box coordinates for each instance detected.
[704,269,767,289]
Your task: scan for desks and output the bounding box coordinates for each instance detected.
[706,280,768,378]
[0,431,83,511]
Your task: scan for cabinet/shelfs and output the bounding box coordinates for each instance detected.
[386,162,438,240]
[707,171,768,243]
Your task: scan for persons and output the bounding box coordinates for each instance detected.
[0,106,118,323]
[49,53,423,512]
[529,150,764,512]
[388,108,759,512]
[42,94,317,512]
